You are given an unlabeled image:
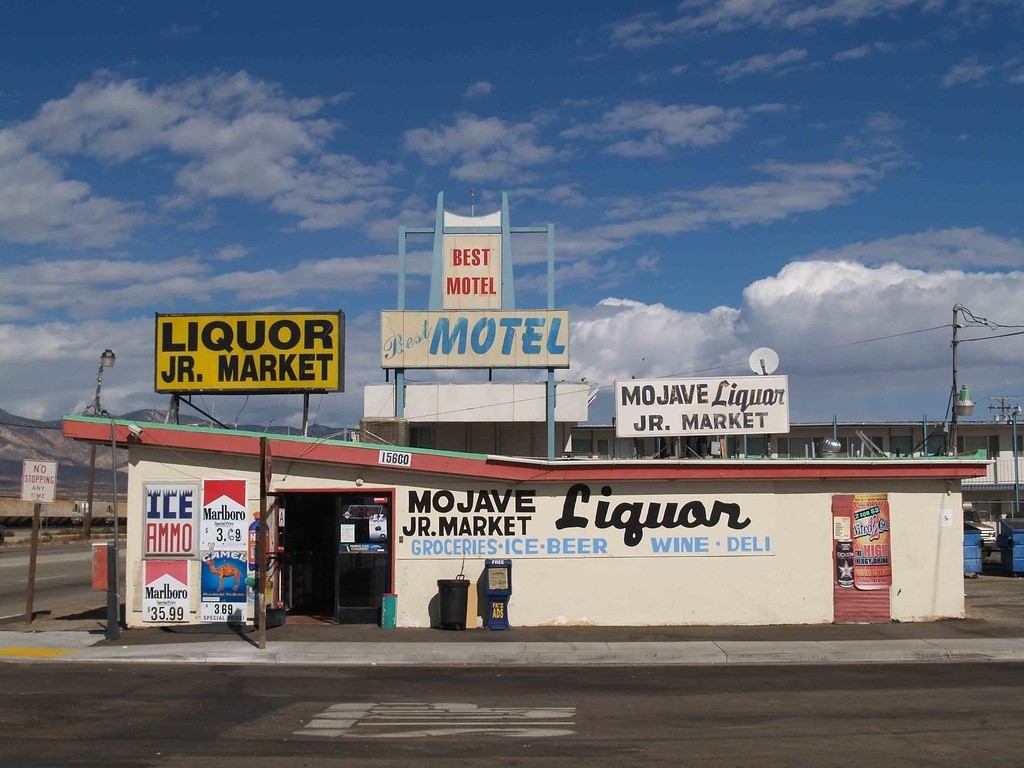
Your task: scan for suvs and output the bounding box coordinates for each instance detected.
[963,511,997,556]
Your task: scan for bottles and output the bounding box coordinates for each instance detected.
[248,512,270,571]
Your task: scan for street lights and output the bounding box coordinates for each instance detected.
[84,348,116,541]
[1012,404,1023,512]
[951,383,974,458]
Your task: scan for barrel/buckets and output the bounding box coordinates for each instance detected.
[437,579,470,630]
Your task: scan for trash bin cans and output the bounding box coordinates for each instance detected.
[437,574,470,631]
